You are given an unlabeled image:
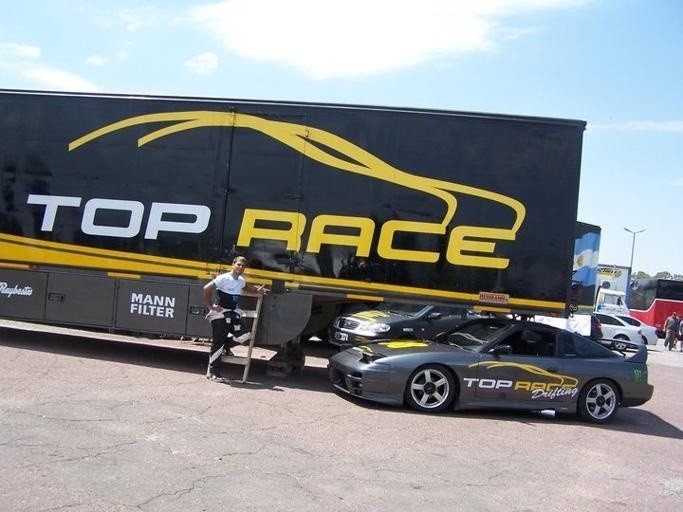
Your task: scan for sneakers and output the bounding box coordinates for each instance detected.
[223,347,233,356]
[205,372,227,383]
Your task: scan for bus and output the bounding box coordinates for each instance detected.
[630,277,682,340]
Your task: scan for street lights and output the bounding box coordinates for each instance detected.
[624,225,646,305]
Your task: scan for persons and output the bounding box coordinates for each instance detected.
[202,255,271,383]
[662,311,683,353]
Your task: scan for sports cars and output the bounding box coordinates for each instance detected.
[326,318,653,423]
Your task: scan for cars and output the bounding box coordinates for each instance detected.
[593,312,642,351]
[612,314,658,348]
[326,301,510,352]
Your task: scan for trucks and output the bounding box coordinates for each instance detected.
[594,286,629,316]
